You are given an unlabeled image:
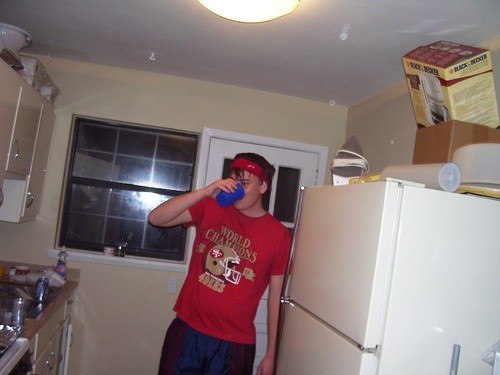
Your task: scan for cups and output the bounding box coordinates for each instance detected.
[216,183,244,206]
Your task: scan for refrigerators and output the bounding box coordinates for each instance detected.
[274,181,499,374]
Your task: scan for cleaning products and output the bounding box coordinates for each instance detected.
[55,245,69,276]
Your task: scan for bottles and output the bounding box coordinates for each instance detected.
[56,246,69,279]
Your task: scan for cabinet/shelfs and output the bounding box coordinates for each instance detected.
[0,277,79,375]
[0,57,57,225]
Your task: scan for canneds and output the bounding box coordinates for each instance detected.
[34,276,49,301]
[11,298,25,326]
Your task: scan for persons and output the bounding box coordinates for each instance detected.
[148,153,291,375]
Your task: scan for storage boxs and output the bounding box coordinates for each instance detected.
[412,119,500,164]
[401,40,500,130]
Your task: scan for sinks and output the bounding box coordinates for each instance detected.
[0,278,61,320]
